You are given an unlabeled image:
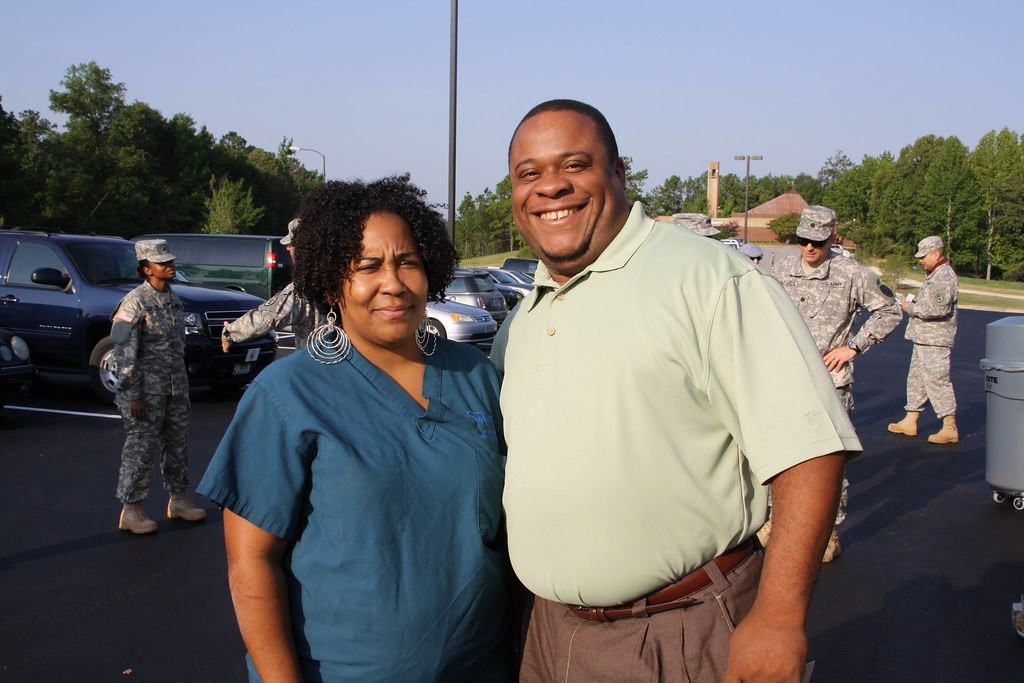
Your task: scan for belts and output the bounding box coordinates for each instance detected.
[568,534,763,622]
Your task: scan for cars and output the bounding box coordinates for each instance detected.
[830,243,858,259]
[720,236,744,249]
[419,256,540,347]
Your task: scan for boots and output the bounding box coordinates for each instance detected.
[119,503,159,534]
[757,519,771,548]
[166,498,207,521]
[888,412,921,435]
[822,527,842,567]
[928,416,959,444]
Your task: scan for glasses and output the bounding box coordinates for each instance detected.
[750,256,763,261]
[798,236,828,247]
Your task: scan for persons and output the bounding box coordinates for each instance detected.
[112,238,207,534]
[196,173,512,683]
[671,213,721,239]
[888,236,959,444]
[222,217,326,353]
[756,205,903,562]
[486,99,864,683]
[740,243,764,265]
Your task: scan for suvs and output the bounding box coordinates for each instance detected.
[0,228,278,409]
[128,232,296,303]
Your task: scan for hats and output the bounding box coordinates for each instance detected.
[135,239,179,263]
[672,213,721,236]
[796,205,837,241]
[279,218,302,245]
[741,244,763,257]
[914,236,944,258]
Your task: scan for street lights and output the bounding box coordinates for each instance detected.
[733,154,764,245]
[289,146,328,183]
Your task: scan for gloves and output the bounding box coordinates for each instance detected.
[221,321,233,353]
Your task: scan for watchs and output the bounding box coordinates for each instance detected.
[848,341,861,354]
